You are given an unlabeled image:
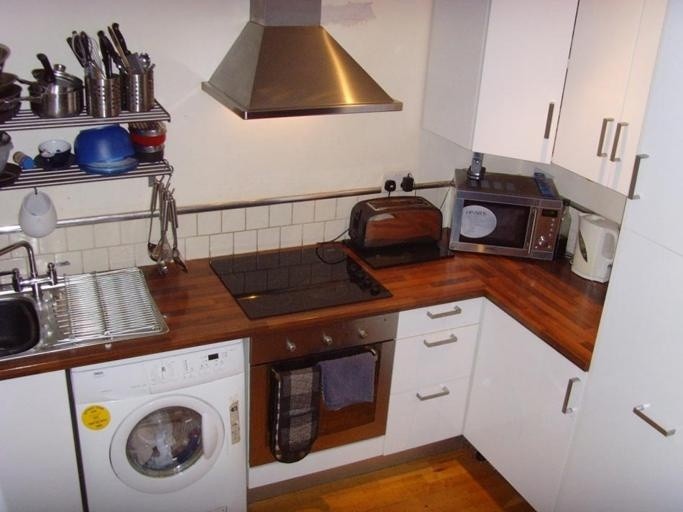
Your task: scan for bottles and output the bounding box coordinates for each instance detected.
[13,151,33,170]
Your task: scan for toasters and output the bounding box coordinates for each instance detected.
[348,195,443,258]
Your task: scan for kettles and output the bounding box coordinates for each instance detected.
[571,213,620,283]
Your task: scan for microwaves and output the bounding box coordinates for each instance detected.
[447,168,564,261]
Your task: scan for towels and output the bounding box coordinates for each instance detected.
[320,352,376,411]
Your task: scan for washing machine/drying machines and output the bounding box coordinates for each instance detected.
[71,340,248,512]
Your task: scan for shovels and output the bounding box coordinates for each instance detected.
[169,198,188,274]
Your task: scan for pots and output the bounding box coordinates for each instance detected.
[3,53,86,120]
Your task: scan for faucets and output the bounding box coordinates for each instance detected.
[0,241,57,299]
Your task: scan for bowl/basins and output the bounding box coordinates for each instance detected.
[37,138,72,167]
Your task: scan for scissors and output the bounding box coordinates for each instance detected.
[73,30,90,68]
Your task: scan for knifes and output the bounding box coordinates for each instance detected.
[111,22,146,74]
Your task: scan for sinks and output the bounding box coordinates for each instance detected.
[0,293,42,358]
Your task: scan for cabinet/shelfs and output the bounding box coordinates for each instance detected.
[422,0,579,163]
[558,0,683,512]
[246,296,485,504]
[0,103,173,191]
[462,301,587,512]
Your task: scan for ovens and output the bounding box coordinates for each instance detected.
[248,311,400,468]
